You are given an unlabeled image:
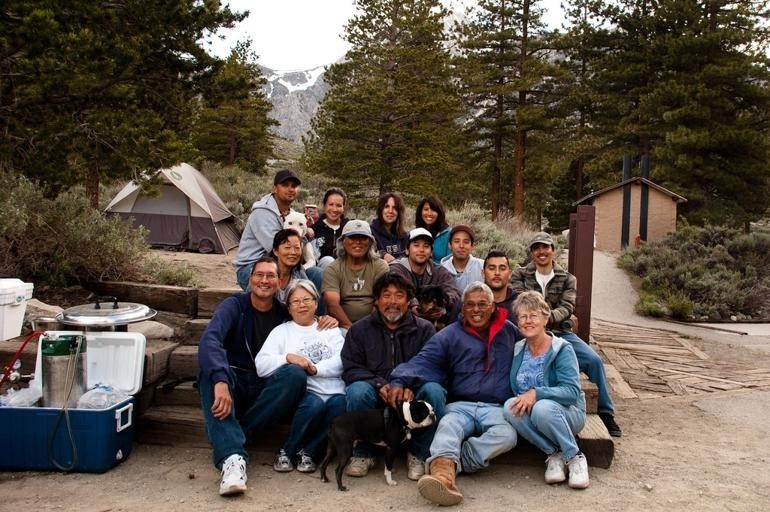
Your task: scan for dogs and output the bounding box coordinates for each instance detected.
[317,399,437,491]
[416,286,452,332]
[278,207,317,271]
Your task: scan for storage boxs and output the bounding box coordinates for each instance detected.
[0,330,147,475]
[0,277,34,343]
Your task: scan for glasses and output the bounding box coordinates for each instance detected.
[463,301,491,309]
[519,313,545,321]
[288,296,314,305]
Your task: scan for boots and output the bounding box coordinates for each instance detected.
[418,457,463,506]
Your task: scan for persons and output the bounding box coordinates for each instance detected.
[510,230,623,438]
[196,257,339,497]
[233,187,590,505]
[234,168,301,291]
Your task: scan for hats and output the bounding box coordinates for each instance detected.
[408,228,434,246]
[336,220,374,244]
[274,170,301,186]
[449,224,475,241]
[528,232,555,251]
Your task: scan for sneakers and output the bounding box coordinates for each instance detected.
[296,446,316,472]
[345,457,374,477]
[407,451,425,480]
[599,413,622,436]
[219,454,247,496]
[568,452,590,489]
[545,452,567,484]
[273,448,293,472]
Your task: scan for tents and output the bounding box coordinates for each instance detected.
[100,162,246,254]
